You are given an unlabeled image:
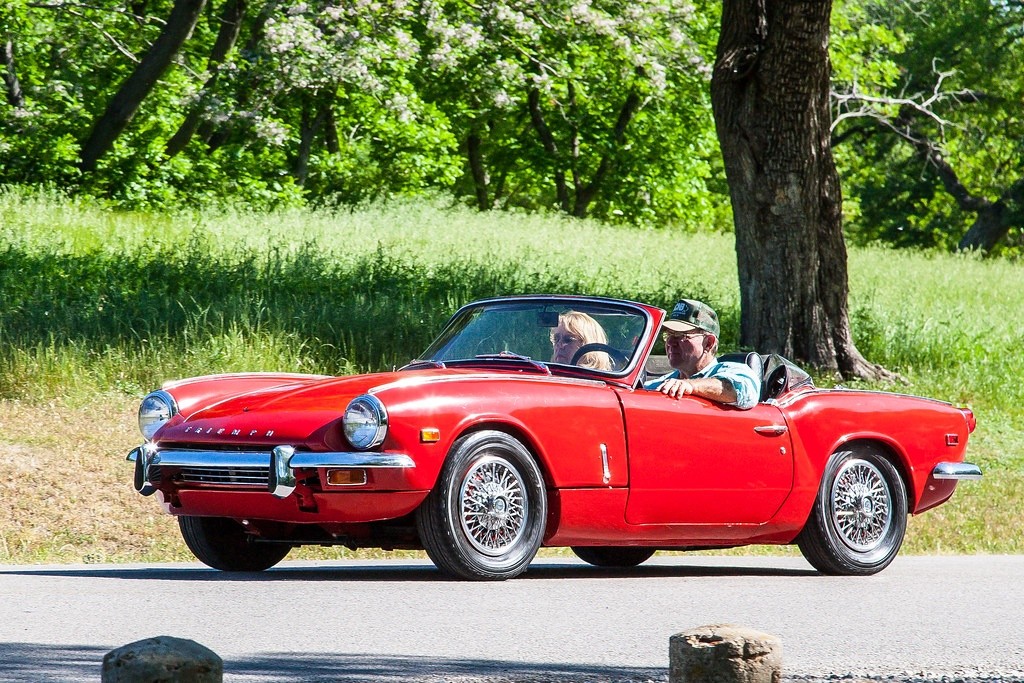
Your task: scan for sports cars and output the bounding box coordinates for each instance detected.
[126,293,983,581]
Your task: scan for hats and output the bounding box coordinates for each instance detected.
[662,298,720,338]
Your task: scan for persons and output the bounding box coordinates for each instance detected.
[549,311,616,370]
[644,299,761,410]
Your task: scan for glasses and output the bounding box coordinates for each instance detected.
[662,330,705,342]
[549,333,577,345]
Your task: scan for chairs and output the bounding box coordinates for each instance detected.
[717,351,763,403]
[608,350,646,385]
